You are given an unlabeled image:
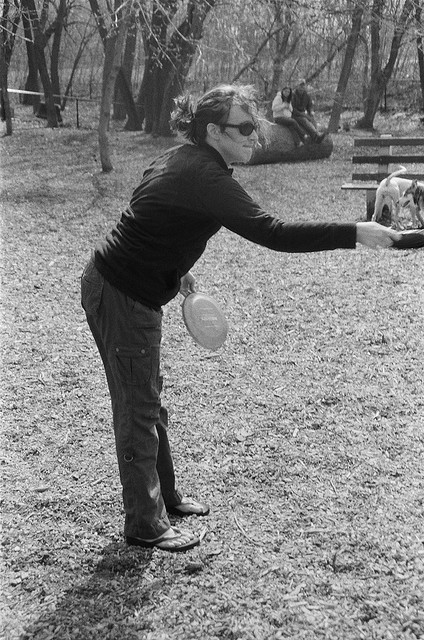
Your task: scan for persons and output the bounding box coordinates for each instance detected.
[292,78,326,144]
[79,83,403,553]
[270,85,311,148]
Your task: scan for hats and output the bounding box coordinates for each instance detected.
[298,78,305,85]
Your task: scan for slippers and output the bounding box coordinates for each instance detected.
[165,496,210,517]
[125,525,200,552]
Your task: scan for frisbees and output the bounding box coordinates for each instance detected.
[182,292,228,349]
[390,230,424,249]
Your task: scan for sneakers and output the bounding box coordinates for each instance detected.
[316,132,325,143]
[304,135,310,145]
[297,142,304,148]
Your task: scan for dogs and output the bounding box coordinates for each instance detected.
[371,164,424,231]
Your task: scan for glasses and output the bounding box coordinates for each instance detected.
[213,120,258,136]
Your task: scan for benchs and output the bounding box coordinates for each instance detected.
[341,134,424,222]
